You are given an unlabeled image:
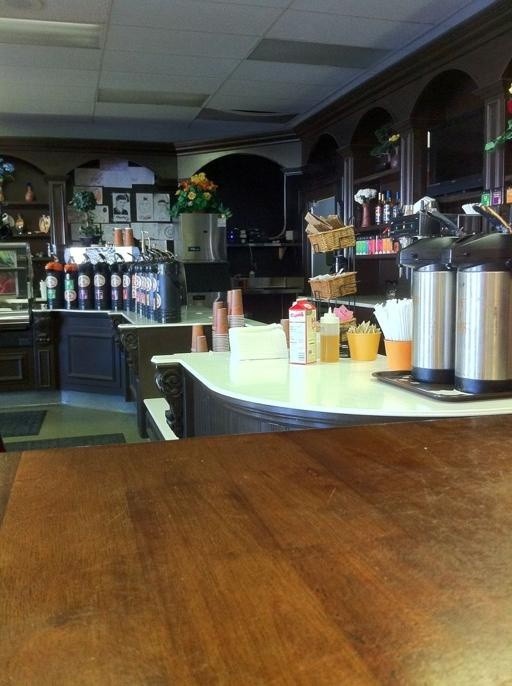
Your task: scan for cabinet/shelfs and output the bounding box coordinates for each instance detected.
[354,166,400,233]
[0,201,52,262]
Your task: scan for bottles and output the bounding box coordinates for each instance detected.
[318,306,341,362]
[355,234,400,256]
[43,247,182,324]
[375,189,401,226]
[112,226,135,246]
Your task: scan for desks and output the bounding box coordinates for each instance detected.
[0,413,512,686]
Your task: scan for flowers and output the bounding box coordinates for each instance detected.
[167,171,233,221]
[367,127,404,157]
[354,188,377,205]
[0,159,15,186]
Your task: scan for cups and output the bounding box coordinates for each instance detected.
[384,338,412,372]
[211,300,230,352]
[345,331,382,360]
[190,323,208,352]
[227,288,247,327]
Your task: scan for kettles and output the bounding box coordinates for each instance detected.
[397,235,459,383]
[439,230,512,393]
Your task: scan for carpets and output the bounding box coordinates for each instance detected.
[0,410,47,437]
[3,433,127,453]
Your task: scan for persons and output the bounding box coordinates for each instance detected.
[114,195,128,214]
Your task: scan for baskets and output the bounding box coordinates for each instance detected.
[340,317,357,342]
[307,224,356,253]
[308,271,357,299]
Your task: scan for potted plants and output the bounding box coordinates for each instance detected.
[68,191,104,247]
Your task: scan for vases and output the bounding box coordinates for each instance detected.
[381,147,401,168]
[360,206,372,228]
[25,186,34,202]
[0,186,5,202]
